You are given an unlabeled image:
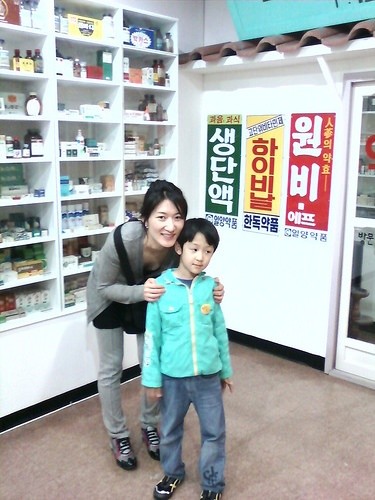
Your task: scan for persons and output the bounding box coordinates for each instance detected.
[142,217,234,500]
[87,178,224,470]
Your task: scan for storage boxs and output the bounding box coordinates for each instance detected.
[60,175,114,196]
[63,256,78,270]
[124,110,145,121]
[0,243,50,326]
[58,101,111,120]
[64,50,112,81]
[124,57,153,86]
[125,167,159,192]
[64,276,87,308]
[124,129,148,156]
[0,0,20,25]
[129,26,154,48]
[68,13,103,40]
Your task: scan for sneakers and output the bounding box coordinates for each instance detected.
[200,489,222,500]
[141,427,161,460]
[110,437,137,469]
[154,470,185,500]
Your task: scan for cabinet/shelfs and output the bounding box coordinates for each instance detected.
[0,0,178,418]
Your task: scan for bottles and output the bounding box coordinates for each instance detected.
[143,94,158,121]
[0,39,43,73]
[157,29,173,51]
[56,49,87,78]
[75,129,86,150]
[7,129,43,158]
[25,92,40,116]
[152,59,170,87]
[102,13,114,38]
[153,137,160,155]
